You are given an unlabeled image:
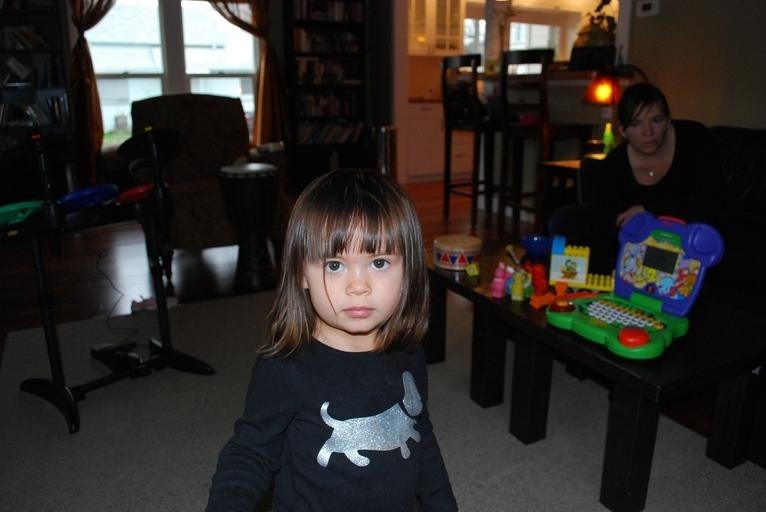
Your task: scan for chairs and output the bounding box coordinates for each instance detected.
[439,55,487,228]
[132,93,282,298]
[480,49,550,234]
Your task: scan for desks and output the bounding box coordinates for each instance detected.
[475,73,617,198]
[425,260,747,511]
[548,157,607,234]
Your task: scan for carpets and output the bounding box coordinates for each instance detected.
[0,290,766,512]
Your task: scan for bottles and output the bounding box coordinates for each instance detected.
[602,122,613,155]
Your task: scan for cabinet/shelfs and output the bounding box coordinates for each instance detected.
[283,0,395,180]
[407,101,475,183]
[0,0,78,174]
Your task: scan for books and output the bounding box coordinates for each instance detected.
[0,0,69,152]
[292,0,365,145]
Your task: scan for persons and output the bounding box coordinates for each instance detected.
[590,81,721,275]
[204,168,459,511]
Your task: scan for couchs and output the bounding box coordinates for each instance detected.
[541,118,765,473]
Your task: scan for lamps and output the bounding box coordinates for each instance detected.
[584,79,623,161]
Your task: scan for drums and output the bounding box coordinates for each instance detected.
[219,163,280,284]
[433,235,482,270]
